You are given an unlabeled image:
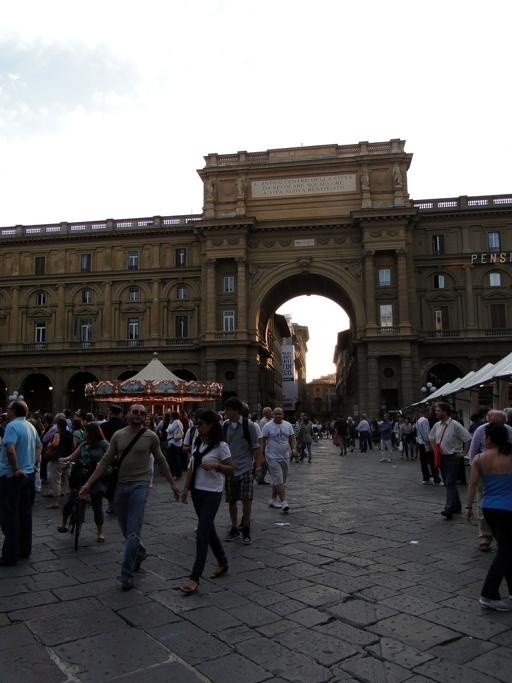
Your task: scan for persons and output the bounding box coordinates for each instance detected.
[78,404,180,590]
[330,402,511,551]
[57,413,95,532]
[180,408,234,593]
[41,403,330,512]
[1,400,45,564]
[466,422,512,613]
[58,422,110,541]
[224,397,262,544]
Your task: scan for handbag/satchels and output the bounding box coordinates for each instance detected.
[69,463,98,490]
[173,421,184,439]
[434,443,441,465]
[104,468,119,500]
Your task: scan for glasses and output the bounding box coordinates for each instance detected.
[133,409,146,415]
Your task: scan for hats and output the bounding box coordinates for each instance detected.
[75,408,87,419]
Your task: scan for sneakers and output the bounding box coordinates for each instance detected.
[436,481,444,485]
[122,588,138,601]
[242,525,253,544]
[479,536,491,551]
[422,480,433,485]
[269,498,281,507]
[478,596,511,612]
[223,526,241,541]
[281,499,290,511]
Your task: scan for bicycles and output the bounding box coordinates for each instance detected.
[56,459,97,550]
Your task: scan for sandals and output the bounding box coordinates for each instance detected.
[210,564,228,578]
[180,579,199,592]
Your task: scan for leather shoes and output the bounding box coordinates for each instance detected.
[98,535,105,541]
[441,510,453,519]
[0,557,17,565]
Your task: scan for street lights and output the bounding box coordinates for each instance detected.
[420,382,437,404]
[8,390,25,402]
